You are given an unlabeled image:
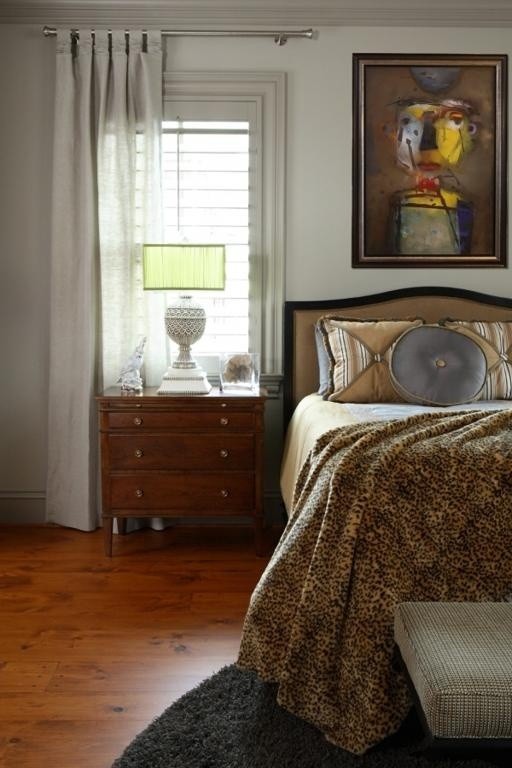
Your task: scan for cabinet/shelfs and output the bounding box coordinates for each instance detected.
[92,384,268,559]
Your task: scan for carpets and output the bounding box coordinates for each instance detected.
[111,661,511,767]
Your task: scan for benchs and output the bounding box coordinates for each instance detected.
[390,596,511,768]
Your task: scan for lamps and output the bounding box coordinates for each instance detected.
[139,240,229,395]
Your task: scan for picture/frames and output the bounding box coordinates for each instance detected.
[350,49,509,273]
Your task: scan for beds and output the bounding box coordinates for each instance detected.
[196,284,511,765]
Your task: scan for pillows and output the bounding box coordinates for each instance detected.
[314,312,512,411]
